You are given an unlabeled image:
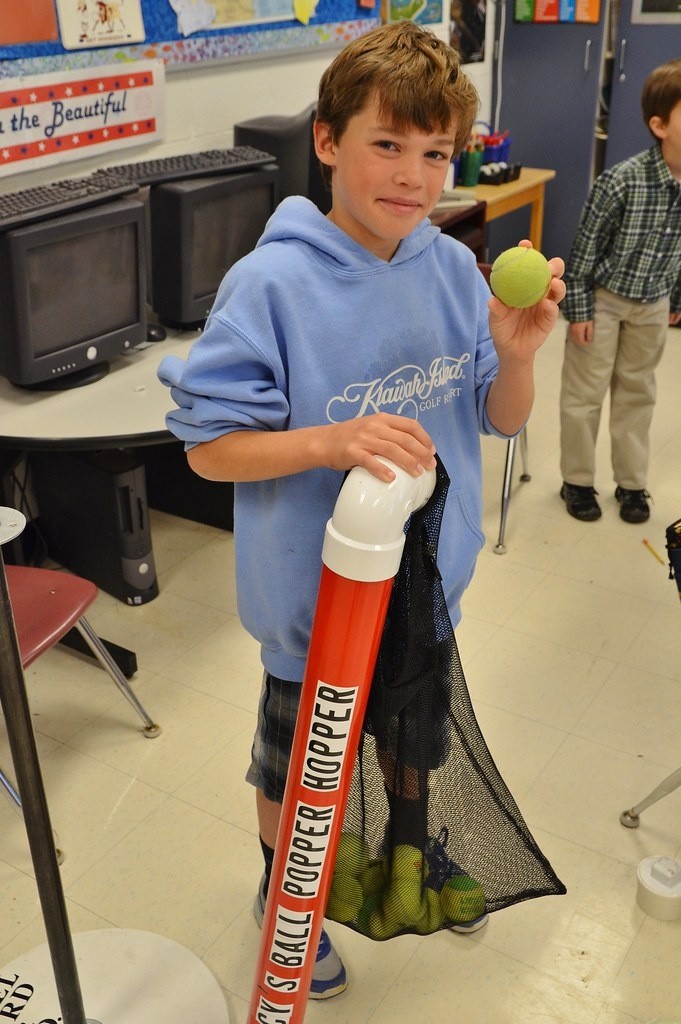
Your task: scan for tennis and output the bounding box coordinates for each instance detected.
[489,247,552,308]
[325,833,486,938]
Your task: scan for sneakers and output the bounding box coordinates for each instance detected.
[616,484,650,523]
[254,869,347,1000]
[376,821,488,932]
[560,480,601,521]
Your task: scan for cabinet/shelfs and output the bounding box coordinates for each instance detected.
[429,190,494,301]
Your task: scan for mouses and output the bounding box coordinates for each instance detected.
[146,323,166,342]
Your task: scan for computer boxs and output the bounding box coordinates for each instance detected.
[26,448,158,606]
[144,440,235,534]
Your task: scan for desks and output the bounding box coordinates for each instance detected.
[0,319,206,611]
[454,167,557,256]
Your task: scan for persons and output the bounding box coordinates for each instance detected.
[559,61,681,523]
[157,22,567,1000]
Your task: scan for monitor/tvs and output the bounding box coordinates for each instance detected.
[147,164,280,332]
[231,98,333,216]
[0,194,152,392]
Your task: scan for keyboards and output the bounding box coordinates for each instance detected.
[92,145,276,187]
[0,172,139,233]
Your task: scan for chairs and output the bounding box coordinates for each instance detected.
[4,562,162,865]
